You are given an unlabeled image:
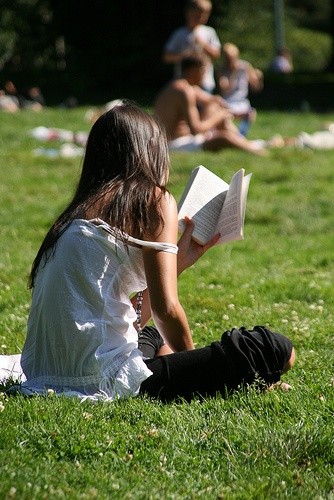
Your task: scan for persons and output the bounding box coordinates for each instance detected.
[270,47,292,74]
[21,106,296,406]
[151,0,268,156]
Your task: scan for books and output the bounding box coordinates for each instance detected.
[177,165,252,246]
